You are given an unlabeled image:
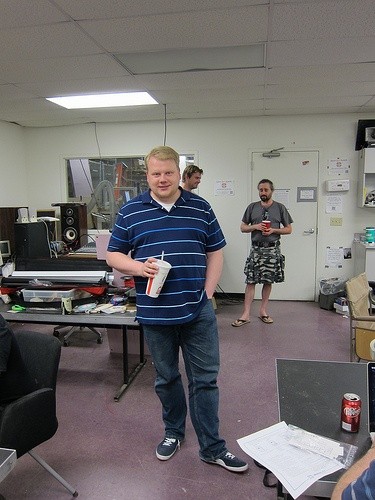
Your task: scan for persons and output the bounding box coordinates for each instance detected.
[0,314,39,420]
[181,165,204,192]
[231,179,293,327]
[331,434,375,500]
[106,146,249,473]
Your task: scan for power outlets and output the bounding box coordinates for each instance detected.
[329,218,343,226]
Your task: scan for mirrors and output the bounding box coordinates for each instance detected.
[63,154,199,230]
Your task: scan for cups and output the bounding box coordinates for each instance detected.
[261,220,272,235]
[366,229,375,244]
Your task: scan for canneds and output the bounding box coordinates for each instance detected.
[340,392,361,433]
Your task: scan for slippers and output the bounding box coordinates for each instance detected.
[258,315,274,323]
[231,318,251,327]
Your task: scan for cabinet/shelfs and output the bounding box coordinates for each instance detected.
[351,241,375,284]
[357,148,375,208]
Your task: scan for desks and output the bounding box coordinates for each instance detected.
[275,357,372,500]
[0,303,147,403]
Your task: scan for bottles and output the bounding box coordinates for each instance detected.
[145,259,172,298]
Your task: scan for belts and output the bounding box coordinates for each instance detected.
[251,240,280,247]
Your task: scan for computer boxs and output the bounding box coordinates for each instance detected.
[15,221,50,258]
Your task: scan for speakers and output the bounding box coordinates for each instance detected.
[18,206,37,223]
[51,202,88,251]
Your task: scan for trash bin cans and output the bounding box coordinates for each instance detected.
[318,278,347,312]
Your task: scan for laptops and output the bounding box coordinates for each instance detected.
[367,362,375,442]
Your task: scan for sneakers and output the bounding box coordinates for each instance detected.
[200,451,249,471]
[156,437,182,460]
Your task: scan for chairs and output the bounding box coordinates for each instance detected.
[0,331,78,498]
[345,272,375,362]
